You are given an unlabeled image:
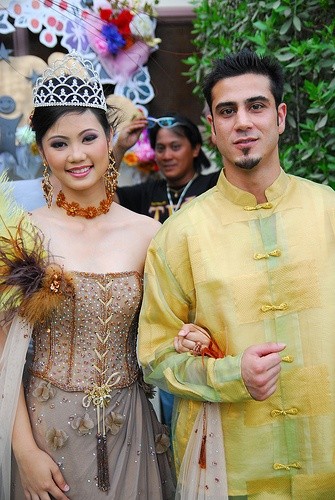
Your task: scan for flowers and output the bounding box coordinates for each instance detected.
[98,4,157,56]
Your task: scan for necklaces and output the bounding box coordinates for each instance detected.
[56,186,113,219]
[166,172,199,214]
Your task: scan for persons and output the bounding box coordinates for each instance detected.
[0,75,211,500]
[136,49,335,500]
[109,113,223,224]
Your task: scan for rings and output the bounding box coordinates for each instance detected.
[193,342,201,351]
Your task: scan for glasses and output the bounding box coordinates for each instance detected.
[145,117,185,129]
